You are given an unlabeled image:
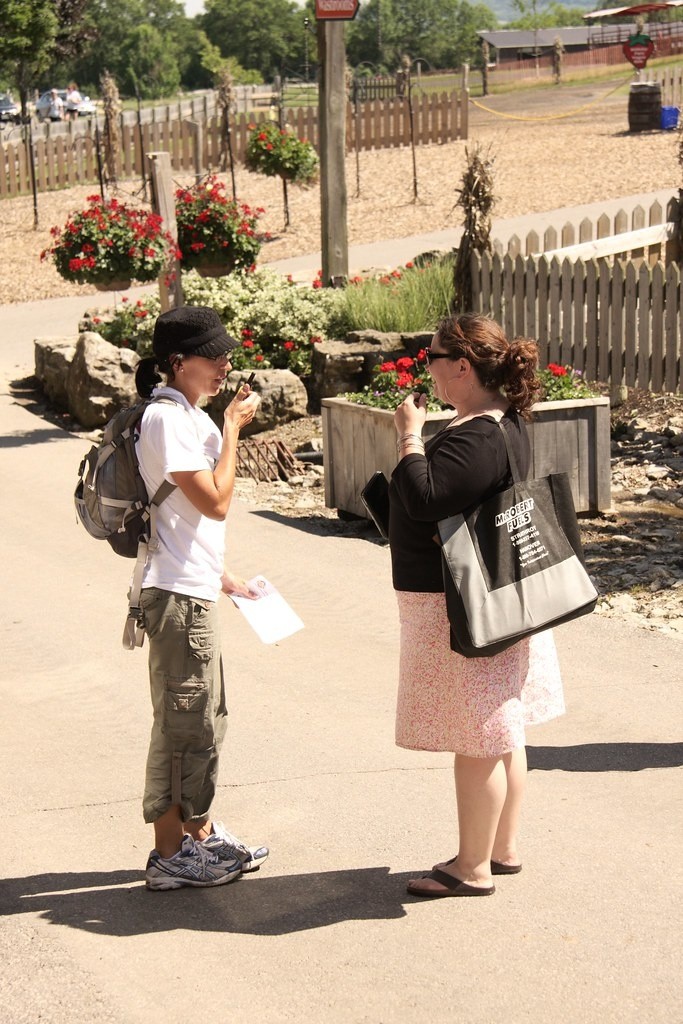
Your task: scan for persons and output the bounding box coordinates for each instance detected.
[360,314,566,896]
[64,81,82,120]
[127,306,268,891]
[48,88,64,122]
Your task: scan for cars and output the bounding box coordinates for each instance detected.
[36,90,97,123]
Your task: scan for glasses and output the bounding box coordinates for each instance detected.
[190,348,234,364]
[426,346,457,366]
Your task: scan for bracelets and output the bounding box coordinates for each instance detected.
[396,433,425,453]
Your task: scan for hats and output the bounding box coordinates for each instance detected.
[152,306,239,358]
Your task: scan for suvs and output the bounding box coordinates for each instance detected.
[0,95,21,125]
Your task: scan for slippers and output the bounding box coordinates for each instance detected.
[407,868,495,897]
[432,854,523,874]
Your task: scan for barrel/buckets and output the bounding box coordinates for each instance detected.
[660,105,679,130]
[627,81,662,131]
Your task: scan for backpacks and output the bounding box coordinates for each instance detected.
[73,396,179,557]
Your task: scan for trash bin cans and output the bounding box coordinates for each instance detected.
[628,81,662,132]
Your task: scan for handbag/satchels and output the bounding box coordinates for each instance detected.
[427,411,597,660]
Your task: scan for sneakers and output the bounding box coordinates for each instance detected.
[145,832,242,891]
[200,821,269,871]
